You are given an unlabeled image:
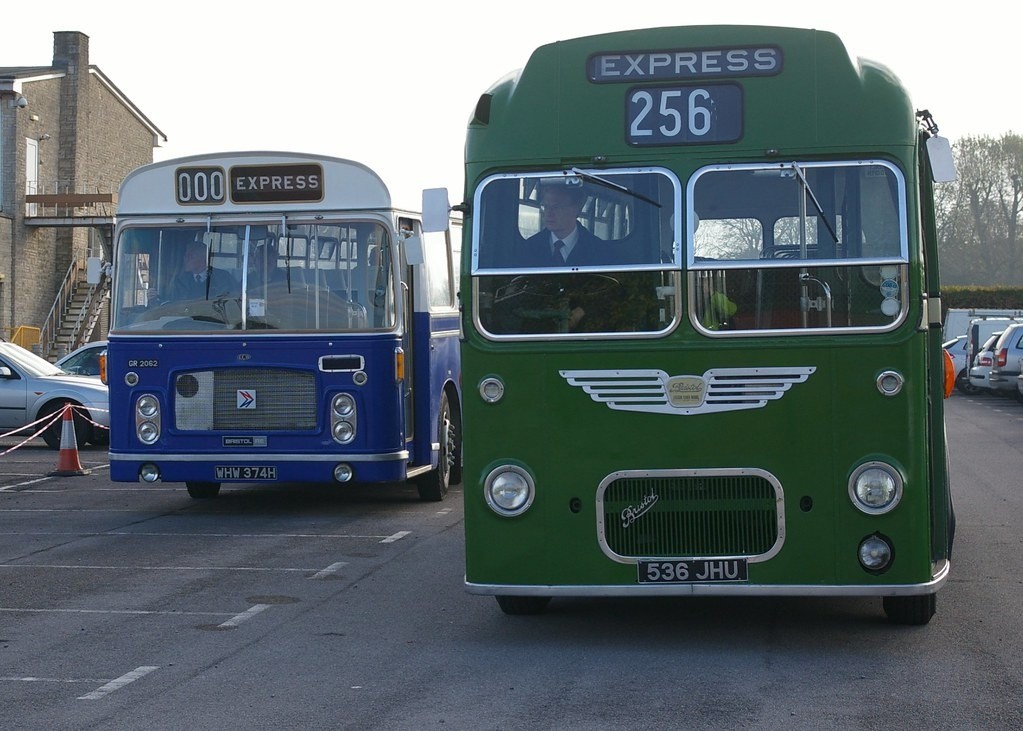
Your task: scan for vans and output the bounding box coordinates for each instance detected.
[941,309,1023,344]
[965,318,1023,379]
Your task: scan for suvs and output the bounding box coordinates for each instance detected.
[942,335,986,395]
[988,324,1023,405]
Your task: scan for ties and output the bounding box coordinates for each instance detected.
[553,240,566,265]
[195,275,201,285]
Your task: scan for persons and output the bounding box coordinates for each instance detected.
[224,245,290,297]
[148,240,236,310]
[520,182,622,331]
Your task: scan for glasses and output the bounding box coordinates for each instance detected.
[539,202,575,213]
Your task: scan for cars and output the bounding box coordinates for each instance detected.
[51,340,110,378]
[0,337,110,451]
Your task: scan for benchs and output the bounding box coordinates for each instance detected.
[754,243,846,329]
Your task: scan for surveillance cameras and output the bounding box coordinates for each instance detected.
[18,97,28,108]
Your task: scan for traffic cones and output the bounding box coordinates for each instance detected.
[45,402,92,477]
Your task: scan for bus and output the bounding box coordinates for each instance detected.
[86,149,463,502]
[421,24,958,629]
